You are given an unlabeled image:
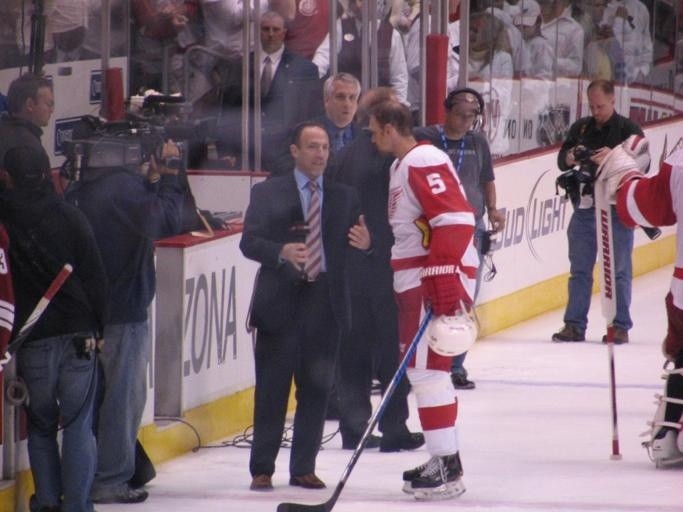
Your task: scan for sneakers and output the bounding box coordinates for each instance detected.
[90,483,149,504]
[601,324,629,345]
[28,493,60,512]
[411,453,464,487]
[402,460,430,482]
[451,373,476,390]
[551,327,587,343]
[652,431,681,462]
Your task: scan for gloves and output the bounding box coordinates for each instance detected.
[417,259,471,316]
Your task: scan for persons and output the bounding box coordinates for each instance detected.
[239,73,506,501]
[614,150,682,468]
[0,0,682,169]
[1,71,184,511]
[551,77,651,344]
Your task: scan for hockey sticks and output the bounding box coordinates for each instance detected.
[277,306,433,512]
[593,135,649,461]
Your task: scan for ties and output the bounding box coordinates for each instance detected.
[302,180,324,283]
[258,57,273,99]
[332,131,347,152]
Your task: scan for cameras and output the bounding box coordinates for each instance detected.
[575,148,596,159]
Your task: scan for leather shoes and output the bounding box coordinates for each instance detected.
[379,428,427,453]
[249,471,273,491]
[288,471,326,489]
[342,433,383,450]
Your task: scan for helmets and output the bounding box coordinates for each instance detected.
[424,304,478,359]
